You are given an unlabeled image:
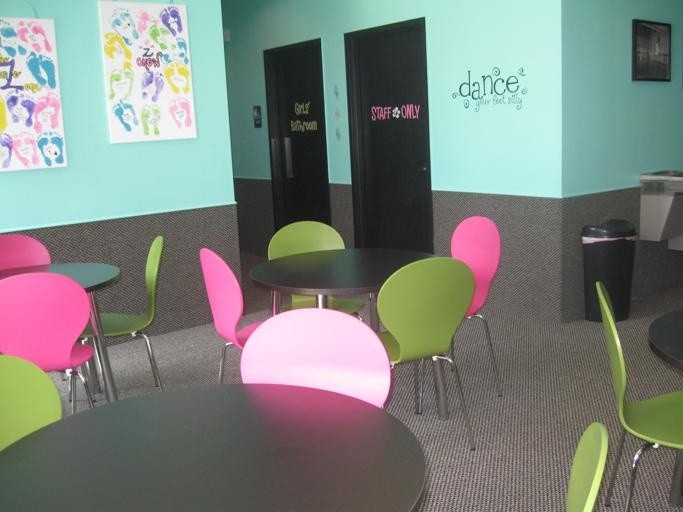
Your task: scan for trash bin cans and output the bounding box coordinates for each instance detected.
[581,218,638,323]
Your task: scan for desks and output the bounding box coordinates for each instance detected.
[249,249,438,310]
[0,379,429,511]
[646,305,683,370]
[3,263,120,402]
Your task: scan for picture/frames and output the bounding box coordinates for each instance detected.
[632,19,671,82]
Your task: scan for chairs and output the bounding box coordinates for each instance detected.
[80,234,165,404]
[372,257,479,451]
[564,421,609,512]
[594,278,681,511]
[414,216,502,415]
[268,220,366,316]
[198,247,264,385]
[0,354,62,451]
[0,272,96,414]
[241,306,394,414]
[1,232,52,272]
